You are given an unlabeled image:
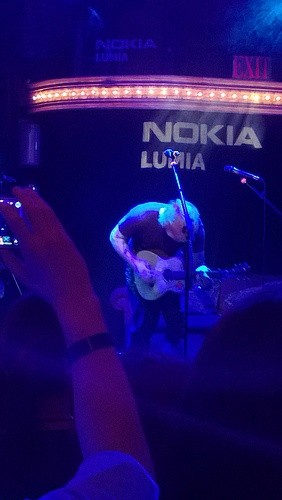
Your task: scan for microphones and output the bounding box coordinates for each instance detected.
[223,166,265,184]
[163,149,181,158]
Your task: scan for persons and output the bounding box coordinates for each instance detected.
[1,186,160,500]
[109,198,207,354]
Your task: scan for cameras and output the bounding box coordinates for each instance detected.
[0,195,31,248]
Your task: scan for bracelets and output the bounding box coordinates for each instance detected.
[68,333,114,365]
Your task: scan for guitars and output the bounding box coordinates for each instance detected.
[133,249,252,301]
